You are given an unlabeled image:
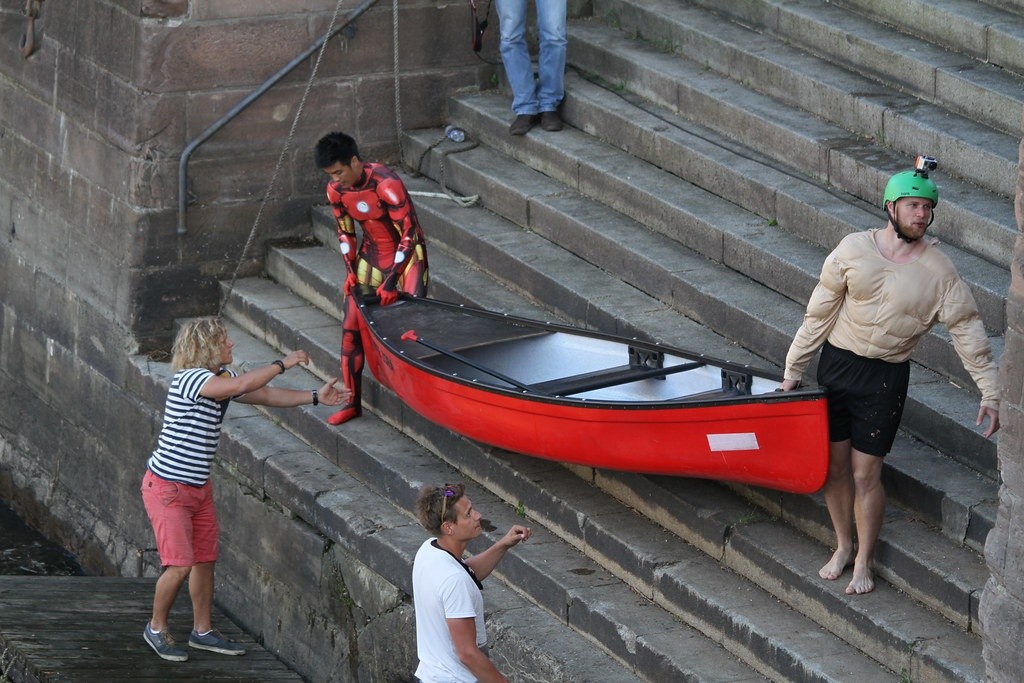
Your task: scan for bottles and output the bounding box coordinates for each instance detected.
[445,126,464,142]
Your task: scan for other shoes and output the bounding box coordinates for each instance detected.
[510,114,539,136]
[539,111,563,131]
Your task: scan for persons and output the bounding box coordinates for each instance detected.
[777,155,1003,594]
[313,130,430,425]
[139,314,355,661]
[494,0,568,136]
[412,479,532,683]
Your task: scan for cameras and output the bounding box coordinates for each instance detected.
[915,155,938,172]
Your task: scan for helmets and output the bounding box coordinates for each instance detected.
[883,156,938,212]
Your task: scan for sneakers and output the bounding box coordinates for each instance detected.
[143,622,188,661]
[189,625,246,655]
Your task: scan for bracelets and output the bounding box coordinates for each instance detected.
[311,389,319,408]
[270,360,286,374]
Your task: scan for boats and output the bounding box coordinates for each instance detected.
[348,281,831,493]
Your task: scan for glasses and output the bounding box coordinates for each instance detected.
[441,483,455,523]
